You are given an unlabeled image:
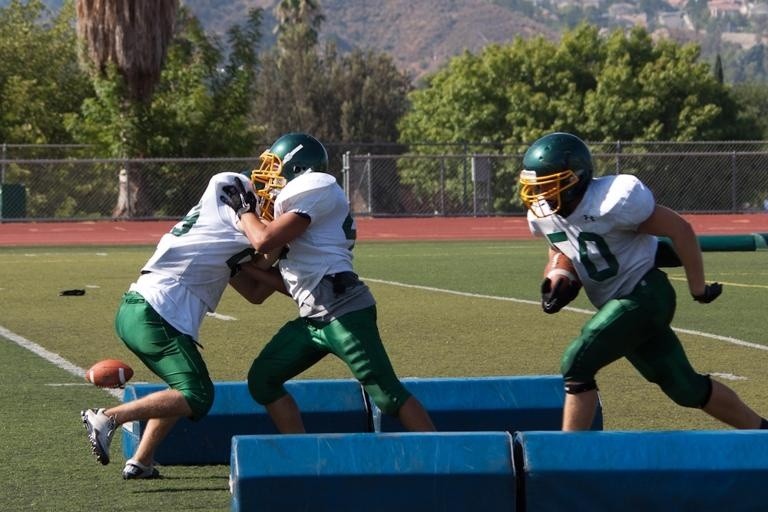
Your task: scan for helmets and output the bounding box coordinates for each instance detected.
[242,132,329,222]
[522,132,593,219]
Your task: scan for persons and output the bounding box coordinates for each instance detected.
[516,133,768,430]
[79,172,283,480]
[218,130,437,434]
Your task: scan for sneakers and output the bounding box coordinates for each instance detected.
[122,458,160,479]
[81,407,119,465]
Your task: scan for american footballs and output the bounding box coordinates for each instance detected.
[543,250,583,297]
[86,360,133,388]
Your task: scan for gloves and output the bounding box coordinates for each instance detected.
[691,282,722,303]
[540,277,579,314]
[220,176,257,220]
